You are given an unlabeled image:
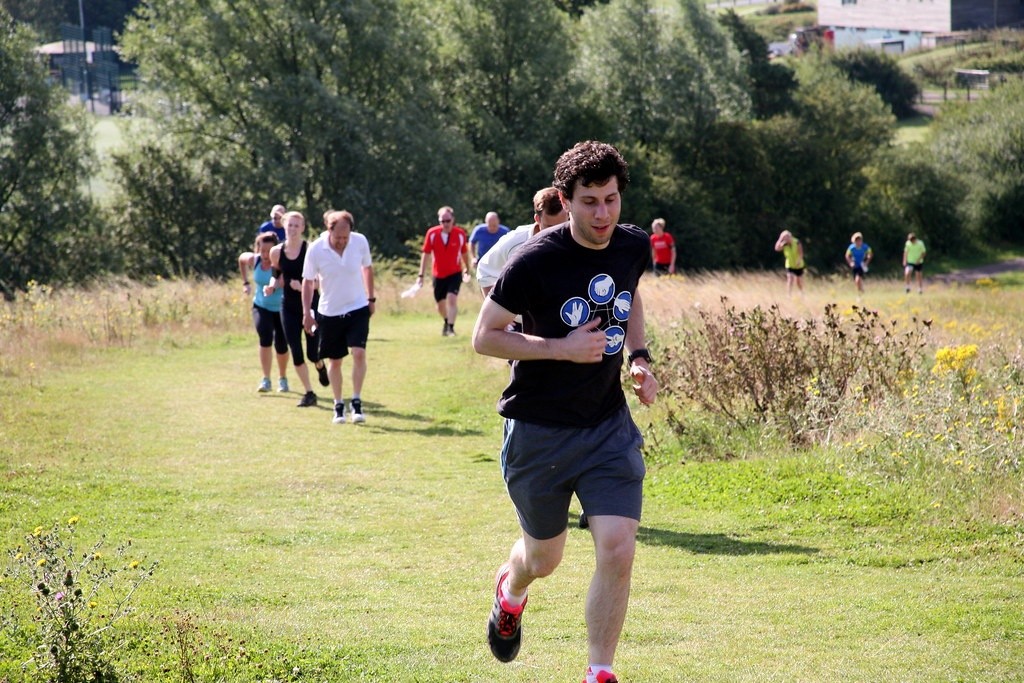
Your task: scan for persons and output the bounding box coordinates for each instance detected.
[468,212,509,268]
[650,218,676,275]
[301,211,375,424]
[260,205,286,242]
[263,211,329,408]
[476,186,569,368]
[903,233,926,294]
[238,232,289,392]
[416,206,470,336]
[775,230,804,290]
[471,141,657,683]
[845,232,872,293]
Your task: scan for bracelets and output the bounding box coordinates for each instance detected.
[273,288,275,293]
[418,275,423,277]
[243,282,249,286]
[369,298,376,302]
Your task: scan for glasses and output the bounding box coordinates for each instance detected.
[437,217,452,223]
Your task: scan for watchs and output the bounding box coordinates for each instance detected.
[628,348,653,365]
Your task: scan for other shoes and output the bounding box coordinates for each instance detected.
[443,321,456,337]
[580,508,591,530]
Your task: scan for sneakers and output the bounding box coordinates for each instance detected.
[315,361,330,386]
[259,379,272,392]
[349,399,364,423]
[486,564,528,662]
[297,393,317,407]
[582,670,620,683]
[279,379,288,393]
[333,401,346,422]
